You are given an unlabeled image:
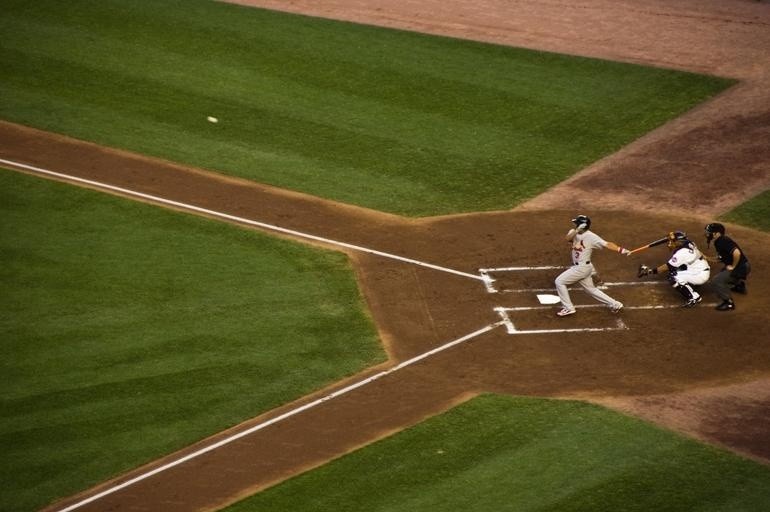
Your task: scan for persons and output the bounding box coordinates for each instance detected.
[699,224,751,312]
[637,231,711,310]
[554,214,631,317]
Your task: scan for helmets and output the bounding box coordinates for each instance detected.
[572,215,591,230]
[705,223,725,240]
[668,231,687,242]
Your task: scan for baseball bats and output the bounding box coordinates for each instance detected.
[631,236,670,254]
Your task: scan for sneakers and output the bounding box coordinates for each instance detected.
[730,281,746,294]
[715,297,735,311]
[681,295,703,308]
[611,301,624,313]
[557,308,576,316]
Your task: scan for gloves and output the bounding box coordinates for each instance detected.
[618,247,631,256]
[576,223,587,233]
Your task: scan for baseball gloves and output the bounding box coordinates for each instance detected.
[637,263,651,278]
[591,274,604,287]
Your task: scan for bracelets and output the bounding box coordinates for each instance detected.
[617,246,625,255]
[652,266,658,275]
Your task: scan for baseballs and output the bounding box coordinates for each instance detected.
[207,117,217,123]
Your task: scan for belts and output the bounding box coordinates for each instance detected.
[575,261,590,265]
